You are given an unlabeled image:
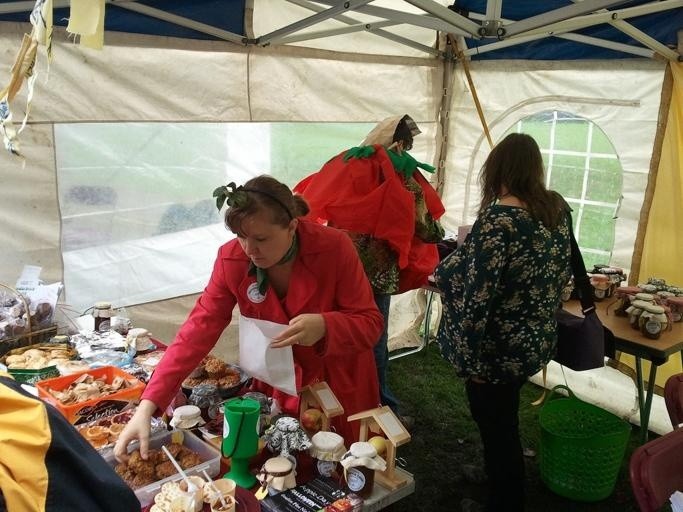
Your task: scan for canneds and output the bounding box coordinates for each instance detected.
[168,405,207,429]
[92,302,115,333]
[561,264,683,340]
[256,416,388,499]
[124,327,154,355]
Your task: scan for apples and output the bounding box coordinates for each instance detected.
[302,409,323,431]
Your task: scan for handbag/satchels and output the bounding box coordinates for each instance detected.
[553,298,615,371]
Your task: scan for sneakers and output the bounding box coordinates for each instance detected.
[401,415,414,430]
[459,463,487,511]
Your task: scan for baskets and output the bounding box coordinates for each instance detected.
[538,385,631,500]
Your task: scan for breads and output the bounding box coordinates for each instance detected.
[4,348,68,369]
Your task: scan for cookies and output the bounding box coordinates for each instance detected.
[113,442,203,512]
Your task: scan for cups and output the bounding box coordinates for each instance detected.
[177,476,236,512]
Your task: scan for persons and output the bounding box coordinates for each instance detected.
[436,132,576,511]
[113,175,382,477]
[291,113,443,416]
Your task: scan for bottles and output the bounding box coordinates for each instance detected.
[126,327,148,358]
[172,404,201,430]
[93,301,111,332]
[560,264,683,340]
[260,416,374,500]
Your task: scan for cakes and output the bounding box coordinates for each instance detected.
[183,355,240,387]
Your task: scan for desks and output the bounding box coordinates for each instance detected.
[413,281,681,443]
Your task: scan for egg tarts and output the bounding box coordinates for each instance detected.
[76,412,133,449]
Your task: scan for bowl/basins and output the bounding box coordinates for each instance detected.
[179,361,249,400]
